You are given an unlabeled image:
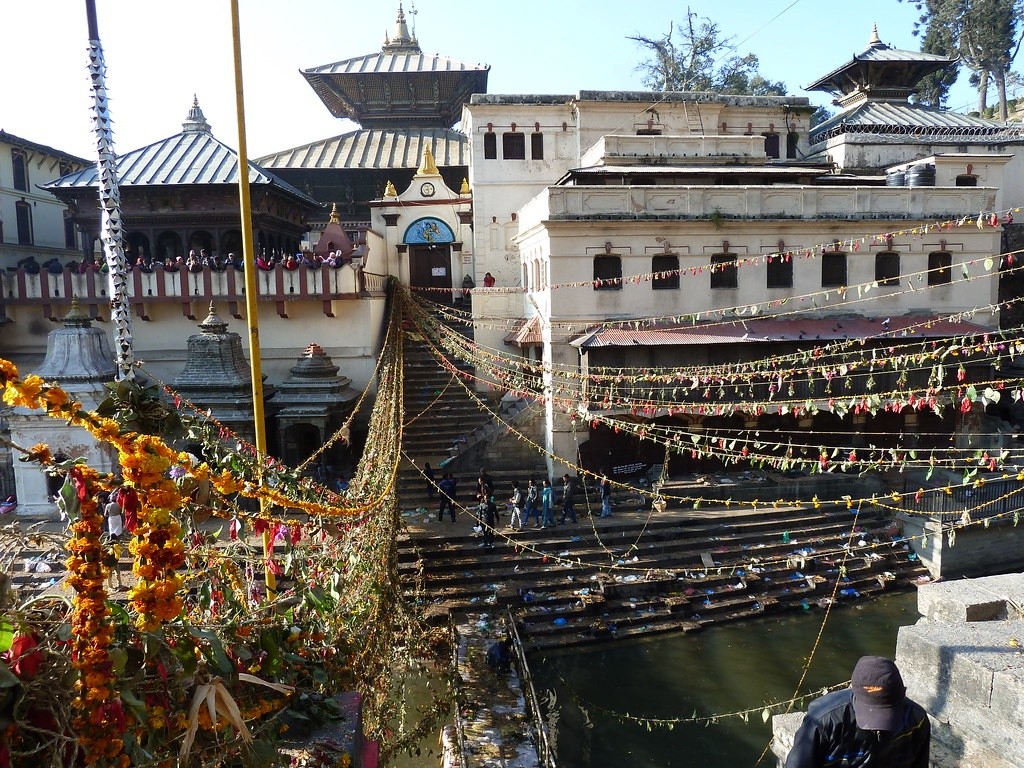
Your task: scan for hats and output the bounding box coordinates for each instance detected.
[851,654,903,731]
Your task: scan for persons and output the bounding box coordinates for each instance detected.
[332,473,350,494]
[420,461,436,497]
[17,248,354,273]
[486,637,515,677]
[508,478,557,530]
[475,467,500,548]
[480,271,497,288]
[599,467,612,518]
[436,472,458,524]
[780,655,931,768]
[558,474,577,525]
[96,494,131,590]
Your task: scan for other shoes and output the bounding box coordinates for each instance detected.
[557,522,565,525]
[549,524,556,527]
[599,514,613,519]
[506,525,514,529]
[451,521,457,525]
[516,527,525,531]
[532,523,540,527]
[479,543,487,547]
[571,520,578,523]
[541,525,547,529]
[436,520,442,524]
[490,543,495,548]
[521,521,528,526]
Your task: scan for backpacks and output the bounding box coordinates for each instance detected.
[515,490,525,509]
